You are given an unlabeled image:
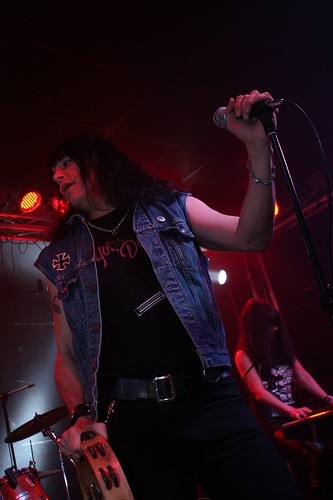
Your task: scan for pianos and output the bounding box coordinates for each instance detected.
[283,405,333,460]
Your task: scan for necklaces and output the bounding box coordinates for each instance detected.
[86,205,131,238]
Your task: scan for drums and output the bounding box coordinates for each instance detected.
[0,467,49,500]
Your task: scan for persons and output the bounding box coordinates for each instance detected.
[235,296,333,500]
[32,88,304,500]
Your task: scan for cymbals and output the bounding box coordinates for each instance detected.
[4,406,68,444]
[37,470,62,479]
[75,431,135,500]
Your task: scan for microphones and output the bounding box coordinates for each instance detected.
[213,98,294,129]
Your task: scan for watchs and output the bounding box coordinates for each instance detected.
[323,394,332,404]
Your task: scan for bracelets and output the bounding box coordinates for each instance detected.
[68,402,97,424]
[245,157,276,185]
